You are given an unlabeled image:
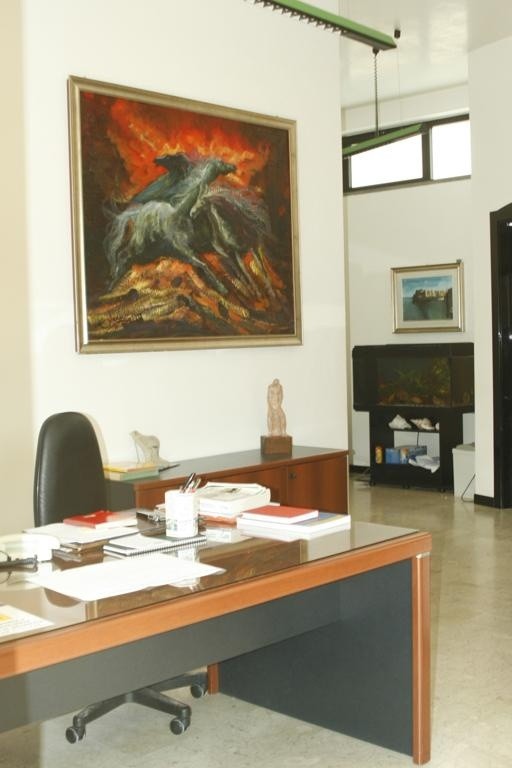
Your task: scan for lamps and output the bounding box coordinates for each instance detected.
[251,0,397,54]
[343,124,427,161]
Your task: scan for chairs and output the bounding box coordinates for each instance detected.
[29,410,207,745]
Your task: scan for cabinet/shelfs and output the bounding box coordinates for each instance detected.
[103,442,353,516]
[368,409,464,489]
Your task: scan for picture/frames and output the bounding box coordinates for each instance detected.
[66,72,302,354]
[388,259,466,334]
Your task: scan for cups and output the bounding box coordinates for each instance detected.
[164,489,199,538]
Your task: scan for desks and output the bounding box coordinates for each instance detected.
[0,503,434,767]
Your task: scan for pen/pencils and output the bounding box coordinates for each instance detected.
[180,472,202,493]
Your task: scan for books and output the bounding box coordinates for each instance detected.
[197,481,351,538]
[1,509,207,567]
[104,461,157,473]
[104,468,159,481]
